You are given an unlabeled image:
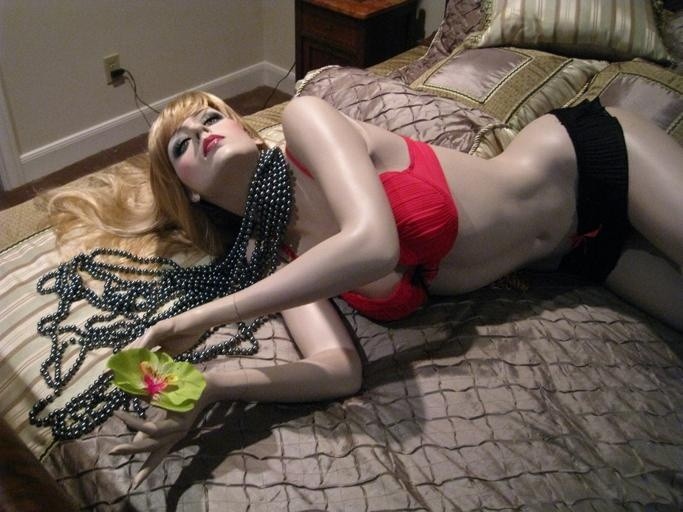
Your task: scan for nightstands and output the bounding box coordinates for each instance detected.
[293,0,422,85]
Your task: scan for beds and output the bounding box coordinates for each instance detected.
[0,43,681,512]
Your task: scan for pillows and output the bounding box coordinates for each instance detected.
[412,0,683,146]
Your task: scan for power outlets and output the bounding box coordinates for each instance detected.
[101,54,122,84]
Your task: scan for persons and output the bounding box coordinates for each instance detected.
[107,89,683,488]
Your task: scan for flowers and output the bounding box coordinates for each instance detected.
[110,346,208,415]
